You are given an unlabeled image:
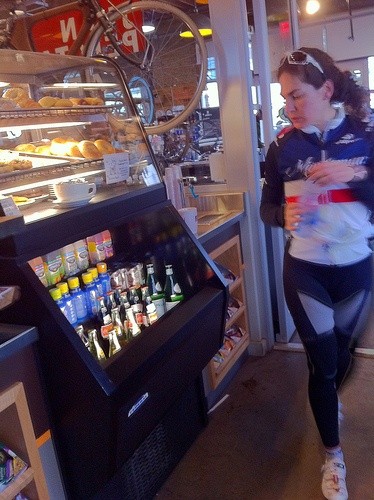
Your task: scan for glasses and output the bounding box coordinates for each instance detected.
[278,50,327,81]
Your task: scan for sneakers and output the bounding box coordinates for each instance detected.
[322,457,349,500]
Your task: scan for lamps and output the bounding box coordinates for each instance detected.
[299,0,320,15]
[180,0,212,38]
[142,17,155,33]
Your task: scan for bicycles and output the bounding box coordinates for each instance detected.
[0,0,221,162]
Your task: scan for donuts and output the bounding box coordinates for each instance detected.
[0,138,114,175]
[0,88,41,110]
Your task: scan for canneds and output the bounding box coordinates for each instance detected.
[28,230,114,288]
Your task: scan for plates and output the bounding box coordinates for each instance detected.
[15,193,50,207]
[50,198,90,208]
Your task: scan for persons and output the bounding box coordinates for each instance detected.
[258,46,374,500]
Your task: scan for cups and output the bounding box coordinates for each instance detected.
[53,181,96,199]
[179,207,198,236]
[160,164,186,209]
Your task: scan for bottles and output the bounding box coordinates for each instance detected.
[97,291,123,358]
[145,264,166,319]
[48,277,87,324]
[75,325,91,352]
[119,287,144,337]
[163,265,184,312]
[88,329,106,361]
[291,175,327,242]
[81,261,113,315]
[141,286,158,324]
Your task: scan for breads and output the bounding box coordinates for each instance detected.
[36,95,102,107]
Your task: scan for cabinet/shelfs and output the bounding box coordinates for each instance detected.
[0,381,49,500]
[0,100,132,194]
[194,234,252,385]
